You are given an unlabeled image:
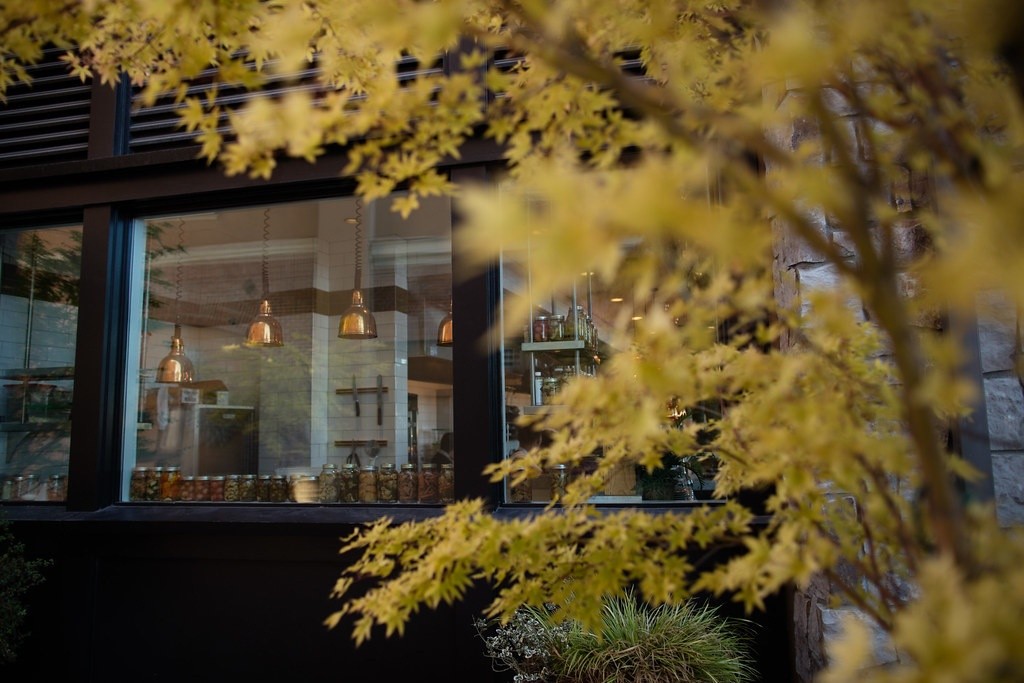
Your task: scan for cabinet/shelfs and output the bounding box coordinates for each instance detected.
[521,188,604,414]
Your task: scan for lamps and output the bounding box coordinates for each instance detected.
[436,298,453,347]
[337,198,378,340]
[154,216,195,384]
[244,207,284,347]
